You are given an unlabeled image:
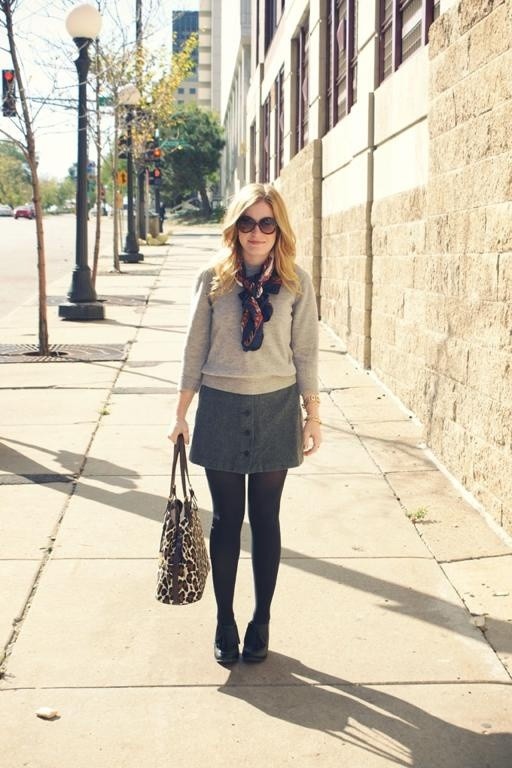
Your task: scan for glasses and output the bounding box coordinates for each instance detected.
[237,216,277,234]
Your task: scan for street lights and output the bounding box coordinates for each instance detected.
[116,80,146,264]
[57,0,106,322]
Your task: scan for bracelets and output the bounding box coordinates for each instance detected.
[304,414,321,425]
[302,395,320,409]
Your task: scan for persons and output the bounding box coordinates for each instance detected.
[167,182,323,664]
[160,201,165,232]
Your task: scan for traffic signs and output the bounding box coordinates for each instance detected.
[99,95,116,105]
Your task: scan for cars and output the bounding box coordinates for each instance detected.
[87,206,108,216]
[46,204,68,214]
[0,204,36,221]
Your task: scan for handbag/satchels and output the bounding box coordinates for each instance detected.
[156,500,210,605]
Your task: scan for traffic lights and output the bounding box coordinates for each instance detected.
[155,149,161,167]
[153,168,161,178]
[2,68,19,118]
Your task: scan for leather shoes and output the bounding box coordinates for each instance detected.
[214,622,240,662]
[242,621,268,661]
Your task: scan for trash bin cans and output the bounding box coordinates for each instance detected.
[143,212,159,238]
